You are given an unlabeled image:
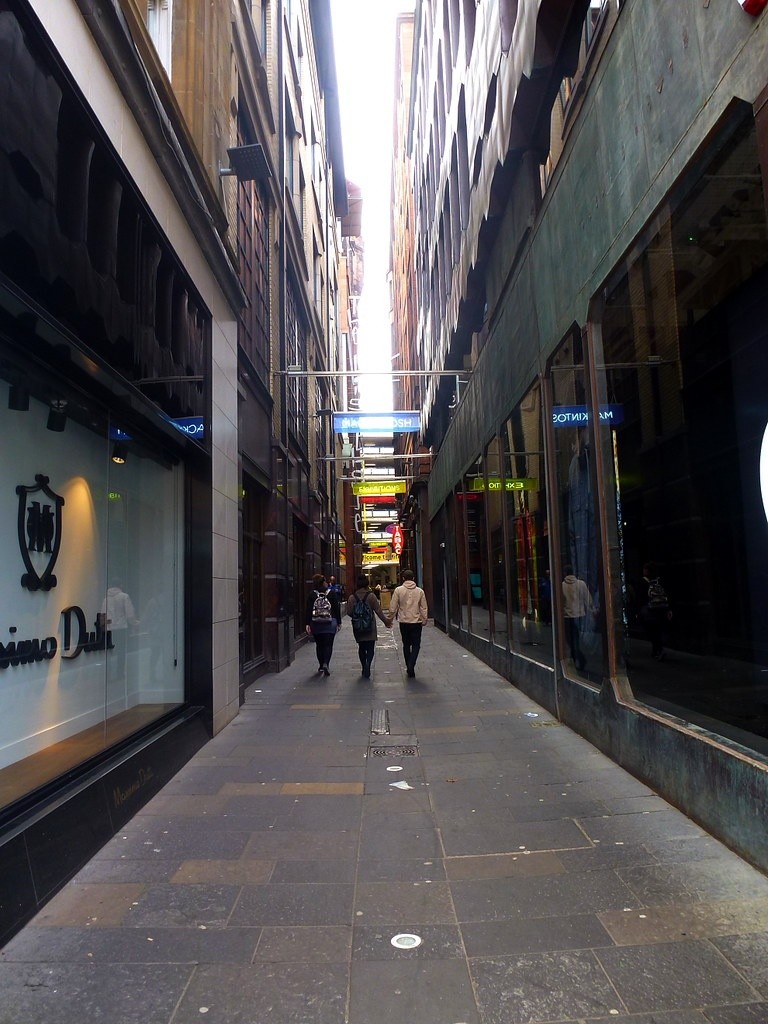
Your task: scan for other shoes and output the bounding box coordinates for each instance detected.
[318,666,323,673]
[322,666,330,677]
[362,664,370,678]
[407,667,415,678]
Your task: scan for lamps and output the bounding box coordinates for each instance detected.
[46,393,68,432]
[111,434,129,465]
[219,143,273,184]
[313,409,332,417]
[8,372,29,411]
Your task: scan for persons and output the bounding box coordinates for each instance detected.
[538,568,551,625]
[100,576,165,684]
[386,570,428,679]
[346,574,392,680]
[562,564,593,671]
[303,572,342,676]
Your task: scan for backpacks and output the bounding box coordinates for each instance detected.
[351,592,374,636]
[312,588,332,621]
